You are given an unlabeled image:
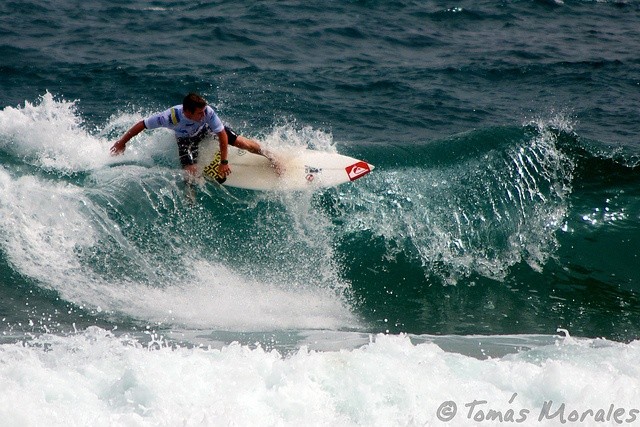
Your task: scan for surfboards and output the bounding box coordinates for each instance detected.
[197,132,376,193]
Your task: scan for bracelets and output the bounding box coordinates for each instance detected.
[221,159,229,164]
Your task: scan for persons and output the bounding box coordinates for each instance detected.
[109,92,287,201]
